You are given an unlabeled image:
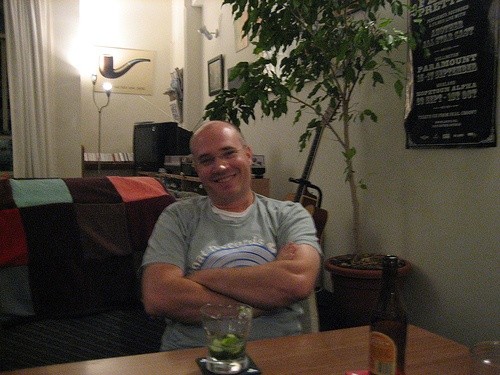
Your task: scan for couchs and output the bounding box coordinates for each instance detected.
[0,174,189,374]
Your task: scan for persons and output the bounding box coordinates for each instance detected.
[141,121,323,353]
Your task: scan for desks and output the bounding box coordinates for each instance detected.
[0,322,492,375]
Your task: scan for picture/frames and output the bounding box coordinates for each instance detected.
[207,54,224,96]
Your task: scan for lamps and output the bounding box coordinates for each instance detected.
[88,74,113,176]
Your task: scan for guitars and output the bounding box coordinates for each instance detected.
[283,96,341,246]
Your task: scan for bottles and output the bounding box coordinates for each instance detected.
[368,255,408,375]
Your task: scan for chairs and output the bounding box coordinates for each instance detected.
[469,341,500,364]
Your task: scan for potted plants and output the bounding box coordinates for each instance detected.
[198,1,428,333]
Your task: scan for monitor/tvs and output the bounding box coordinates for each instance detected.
[132,122,193,173]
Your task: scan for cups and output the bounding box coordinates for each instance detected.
[199,302,254,374]
[467,341,500,375]
